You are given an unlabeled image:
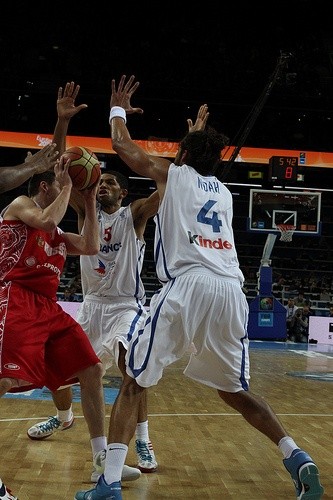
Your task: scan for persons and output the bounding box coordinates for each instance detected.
[294,301,318,344]
[237,251,333,302]
[0,142,60,500]
[284,297,300,342]
[26,82,210,473]
[57,257,83,303]
[74,74,325,500]
[326,306,333,332]
[141,259,164,307]
[0,155,141,482]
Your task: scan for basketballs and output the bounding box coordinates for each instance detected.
[59,146,101,190]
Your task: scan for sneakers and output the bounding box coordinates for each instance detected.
[92,450,141,481]
[76,474,123,500]
[0,479,17,500]
[27,410,74,441]
[283,449,324,499]
[134,439,158,473]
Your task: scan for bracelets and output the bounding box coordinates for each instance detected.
[109,106,127,124]
